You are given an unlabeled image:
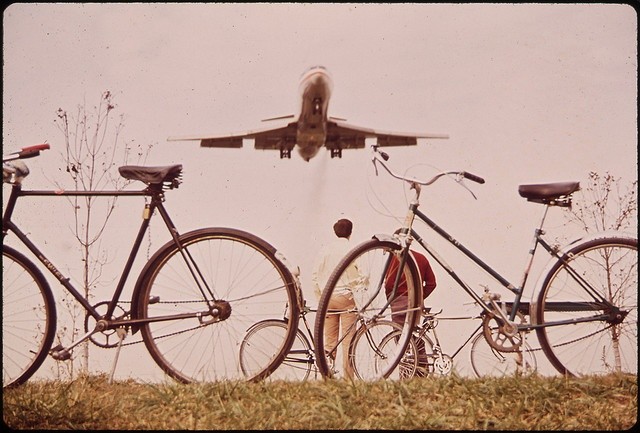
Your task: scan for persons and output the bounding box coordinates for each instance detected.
[384,229,435,377]
[312,218,368,378]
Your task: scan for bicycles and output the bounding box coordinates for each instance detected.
[2,143,301,393]
[312,142,640,382]
[372,282,539,385]
[238,265,420,391]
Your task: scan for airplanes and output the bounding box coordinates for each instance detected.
[164,63,451,160]
